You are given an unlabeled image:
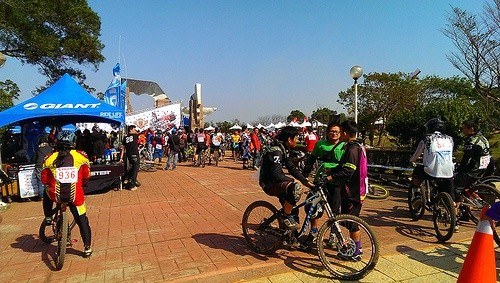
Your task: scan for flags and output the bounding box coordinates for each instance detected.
[104,63,128,130]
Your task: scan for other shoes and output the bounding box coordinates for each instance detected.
[84,246,93,257]
[285,214,363,261]
[44,216,52,226]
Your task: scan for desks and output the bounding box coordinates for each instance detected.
[0,161,126,205]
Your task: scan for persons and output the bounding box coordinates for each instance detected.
[454,117,498,222]
[296,122,347,246]
[0,122,137,257]
[138,126,231,170]
[479,202,500,231]
[233,126,282,176]
[408,118,461,234]
[258,125,316,251]
[325,121,369,260]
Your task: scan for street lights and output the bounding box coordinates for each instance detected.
[350,66,363,125]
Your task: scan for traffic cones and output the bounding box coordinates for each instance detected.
[455,207,497,283]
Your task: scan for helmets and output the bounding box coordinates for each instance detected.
[57,130,78,142]
[424,119,446,132]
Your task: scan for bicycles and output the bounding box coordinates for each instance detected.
[241,179,380,281]
[365,184,391,200]
[34,173,78,272]
[423,158,500,227]
[180,143,226,168]
[407,158,456,240]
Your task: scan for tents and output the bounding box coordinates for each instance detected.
[0,72,127,192]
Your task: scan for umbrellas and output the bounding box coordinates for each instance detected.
[229,119,328,129]
[203,124,219,131]
